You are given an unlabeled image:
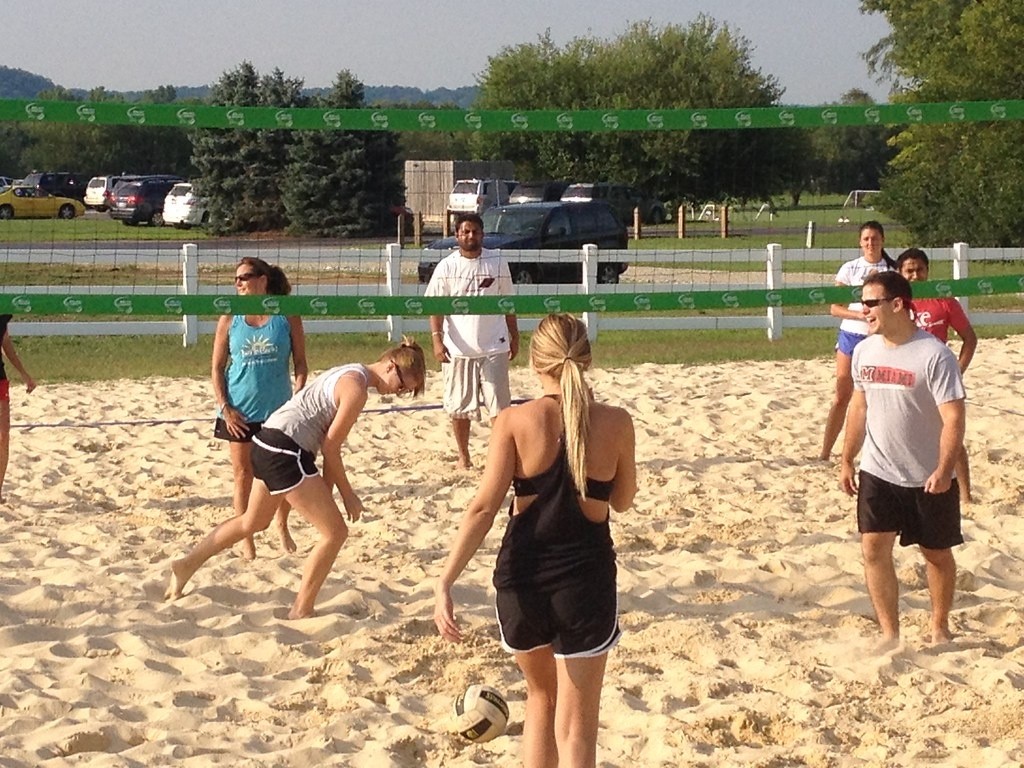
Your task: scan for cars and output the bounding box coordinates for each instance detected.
[163,183,212,230]
[0,186,86,219]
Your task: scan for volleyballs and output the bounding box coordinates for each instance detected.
[454,683,510,743]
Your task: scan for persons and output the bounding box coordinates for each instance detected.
[210,255,309,561]
[817,216,976,505]
[838,270,967,648]
[0,314,38,505]
[432,310,636,767]
[422,215,519,471]
[164,334,428,620]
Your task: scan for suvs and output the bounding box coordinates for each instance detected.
[449,179,522,214]
[418,202,628,285]
[83,175,186,227]
[509,180,570,204]
[0,172,90,205]
[560,182,667,227]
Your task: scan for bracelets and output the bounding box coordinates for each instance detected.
[432,331,442,338]
[221,401,229,415]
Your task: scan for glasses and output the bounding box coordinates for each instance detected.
[860,297,898,308]
[390,360,412,397]
[235,273,258,284]
